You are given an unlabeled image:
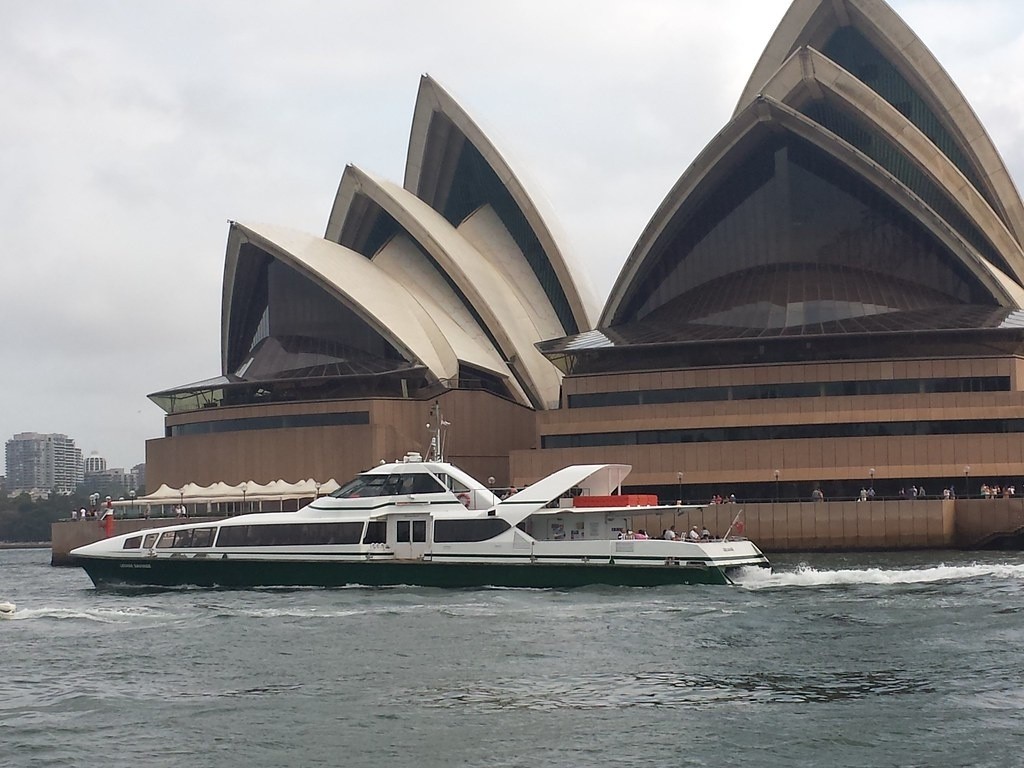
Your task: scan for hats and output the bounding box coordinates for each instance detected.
[731,494,734,498]
[693,526,697,529]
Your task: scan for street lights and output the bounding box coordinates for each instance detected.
[179,488,185,518]
[129,490,136,519]
[90,495,96,516]
[241,485,247,514]
[676,471,683,505]
[773,469,781,503]
[868,468,875,501]
[315,483,321,498]
[963,465,972,499]
[94,493,99,521]
[488,476,496,493]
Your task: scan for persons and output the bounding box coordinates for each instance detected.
[500,484,527,499]
[811,488,824,502]
[899,485,925,499]
[176,504,187,518]
[71,507,96,521]
[981,484,1015,499]
[663,525,710,542]
[860,486,875,501]
[618,528,649,540]
[711,493,736,504]
[943,486,955,500]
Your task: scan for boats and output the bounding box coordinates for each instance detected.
[0,601,19,621]
[62,398,775,592]
